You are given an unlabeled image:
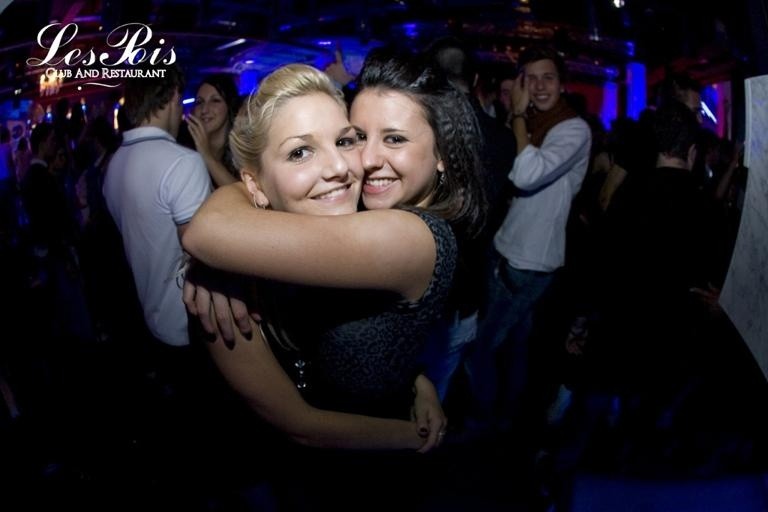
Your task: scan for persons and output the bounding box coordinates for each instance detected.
[0,37,768,510]
